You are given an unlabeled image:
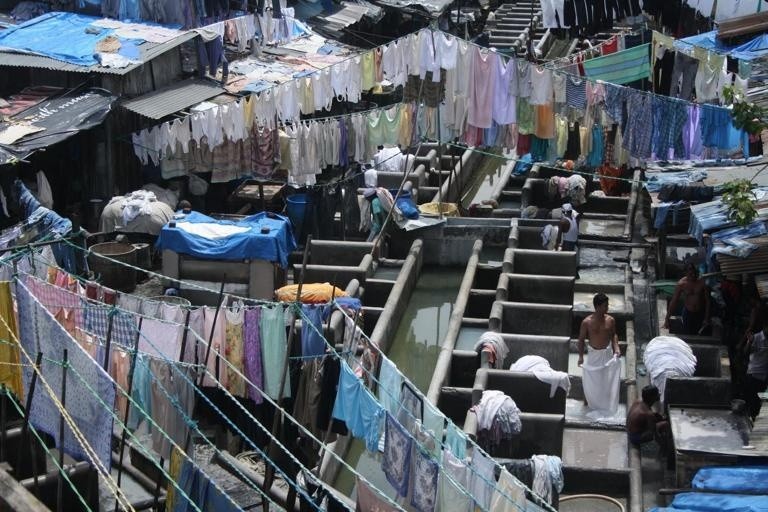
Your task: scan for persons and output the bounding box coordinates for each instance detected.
[577,294,622,406]
[555,202,580,280]
[364,189,385,228]
[661,262,766,422]
[627,385,671,451]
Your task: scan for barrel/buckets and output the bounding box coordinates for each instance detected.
[88,243,138,294]
[132,243,152,271]
[389,189,412,201]
[558,494,625,512]
[287,194,308,227]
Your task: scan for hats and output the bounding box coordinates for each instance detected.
[560,202,572,213]
